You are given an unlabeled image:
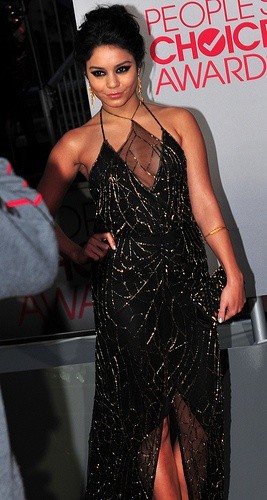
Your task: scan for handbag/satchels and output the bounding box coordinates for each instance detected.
[188,265,246,327]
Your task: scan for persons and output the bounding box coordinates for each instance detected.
[37,4,247,499]
[0,155,60,500]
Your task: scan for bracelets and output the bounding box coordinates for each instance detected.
[204,225,228,240]
